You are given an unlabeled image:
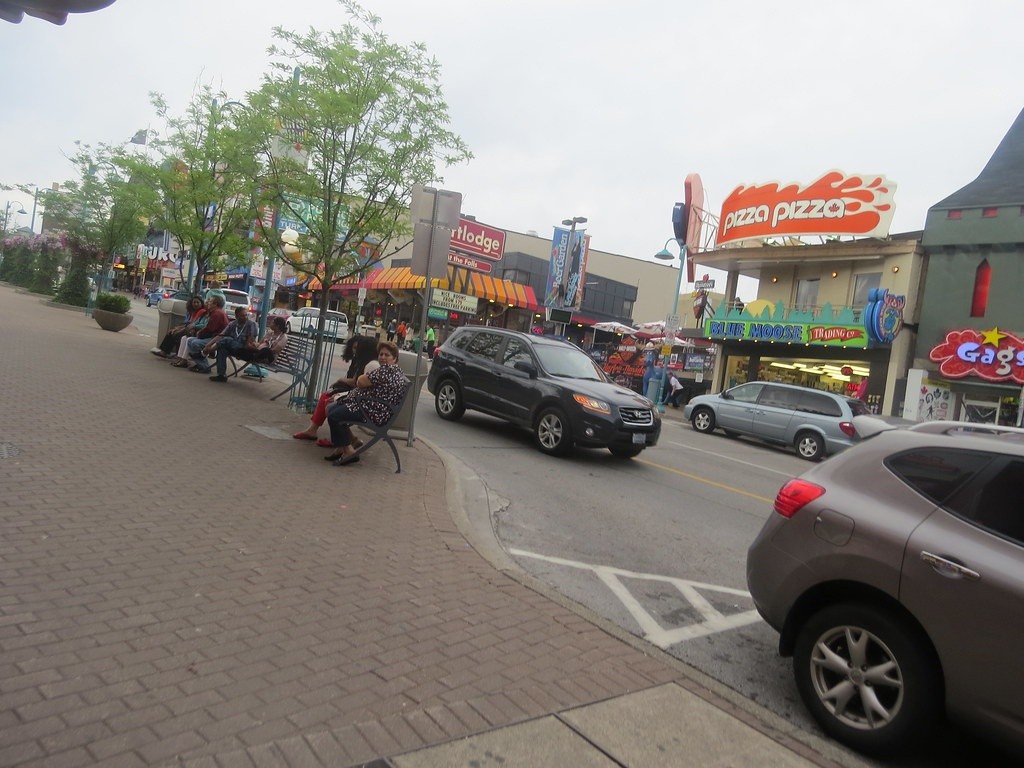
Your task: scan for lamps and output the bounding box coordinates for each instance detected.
[805,364,819,370]
[790,362,818,369]
[842,365,870,372]
[768,361,797,370]
[815,364,841,371]
[853,370,869,376]
[798,368,850,382]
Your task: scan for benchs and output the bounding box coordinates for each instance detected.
[226,330,318,401]
[206,317,238,378]
[332,374,413,474]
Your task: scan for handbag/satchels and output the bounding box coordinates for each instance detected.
[397,332,402,337]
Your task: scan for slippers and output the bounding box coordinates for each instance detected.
[293,431,316,440]
[317,438,334,446]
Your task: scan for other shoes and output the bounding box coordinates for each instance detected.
[210,375,226,382]
[190,364,211,373]
[334,454,359,467]
[171,359,188,366]
[351,437,363,449]
[152,348,168,358]
[189,351,207,361]
[324,449,344,460]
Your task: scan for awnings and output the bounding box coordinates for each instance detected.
[308,265,538,310]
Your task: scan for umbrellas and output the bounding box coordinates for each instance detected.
[651,336,685,347]
[591,321,638,343]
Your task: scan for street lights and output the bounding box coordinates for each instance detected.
[0,199,27,261]
[553,215,588,335]
[651,236,688,414]
[88,160,119,318]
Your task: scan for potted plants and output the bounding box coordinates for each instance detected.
[92,291,134,332]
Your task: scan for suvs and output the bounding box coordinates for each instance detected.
[426,324,663,460]
[284,307,350,344]
[744,412,1024,767]
[204,287,254,321]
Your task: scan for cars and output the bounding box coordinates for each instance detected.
[146,286,207,307]
[681,379,875,463]
[256,306,296,327]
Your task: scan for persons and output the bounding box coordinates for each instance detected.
[375,317,383,339]
[324,341,405,466]
[205,280,226,312]
[237,316,288,365]
[386,319,414,349]
[292,335,381,462]
[201,306,256,383]
[133,285,149,300]
[151,295,229,373]
[667,371,683,407]
[424,324,435,362]
